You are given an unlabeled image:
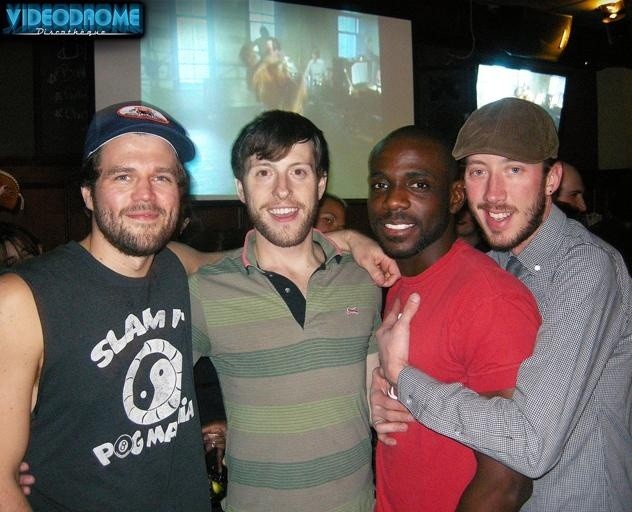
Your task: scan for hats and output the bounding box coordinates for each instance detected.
[82,101,194,168]
[452,98,560,164]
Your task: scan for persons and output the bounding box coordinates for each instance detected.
[239,23,306,114]
[456,207,493,254]
[1,207,44,272]
[0,99,403,512]
[551,157,588,215]
[313,191,350,235]
[202,124,542,512]
[368,97,632,512]
[305,49,327,88]
[186,108,386,512]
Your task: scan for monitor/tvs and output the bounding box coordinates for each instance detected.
[461,48,582,164]
